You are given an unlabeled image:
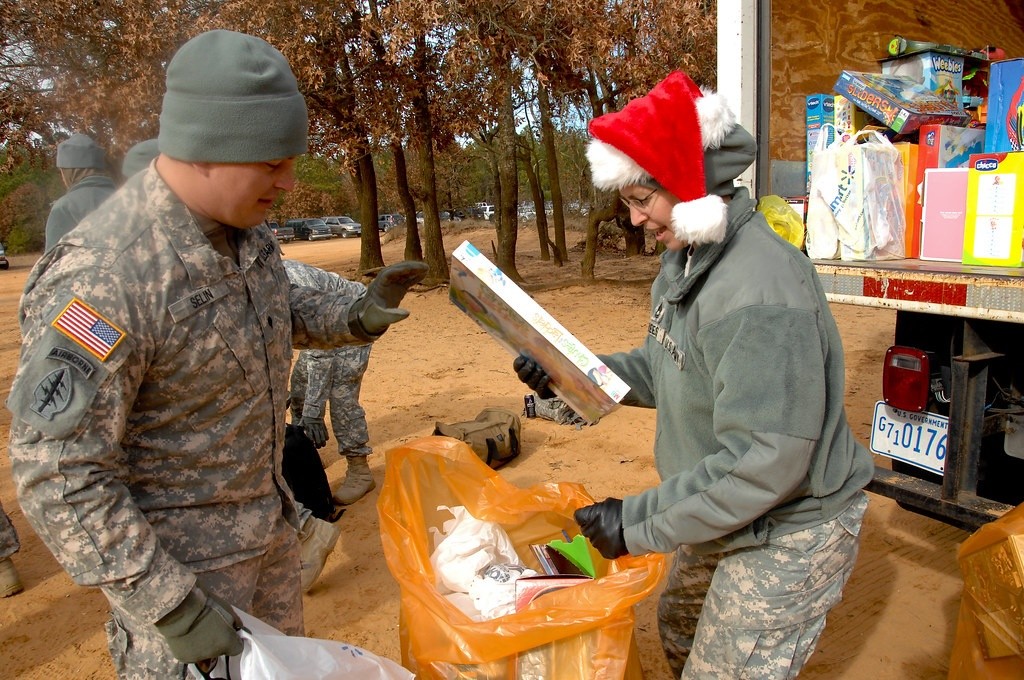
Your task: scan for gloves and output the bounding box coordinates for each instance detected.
[347,261,431,342]
[513,356,558,400]
[573,497,629,559]
[298,416,329,449]
[154,591,244,663]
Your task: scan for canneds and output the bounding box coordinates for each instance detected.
[524,394,535,418]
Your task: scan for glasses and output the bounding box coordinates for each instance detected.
[617,189,658,209]
[194,625,240,680]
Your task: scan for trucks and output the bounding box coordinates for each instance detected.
[710,0,1024,545]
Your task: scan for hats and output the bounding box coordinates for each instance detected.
[157,30,309,162]
[584,69,758,246]
[56,133,105,170]
[122,139,161,177]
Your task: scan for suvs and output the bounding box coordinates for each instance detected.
[320,216,362,239]
[284,217,334,241]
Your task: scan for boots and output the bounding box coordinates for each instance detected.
[333,456,375,505]
[297,515,342,593]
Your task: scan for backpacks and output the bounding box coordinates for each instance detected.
[282,423,346,522]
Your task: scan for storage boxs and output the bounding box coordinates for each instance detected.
[446,240,632,425]
[804,36,1024,272]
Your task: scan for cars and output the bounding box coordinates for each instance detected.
[414,200,495,224]
[378,213,406,232]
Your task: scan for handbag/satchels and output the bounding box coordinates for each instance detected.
[759,123,905,262]
[188,605,416,680]
[431,407,521,469]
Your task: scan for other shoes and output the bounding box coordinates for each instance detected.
[0,559,25,598]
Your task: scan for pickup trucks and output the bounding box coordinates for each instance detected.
[268,221,295,244]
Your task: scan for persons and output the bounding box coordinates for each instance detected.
[123,139,159,177]
[46,134,115,250]
[8,31,428,680]
[513,71,873,679]
[280,259,375,505]
[0,502,24,599]
